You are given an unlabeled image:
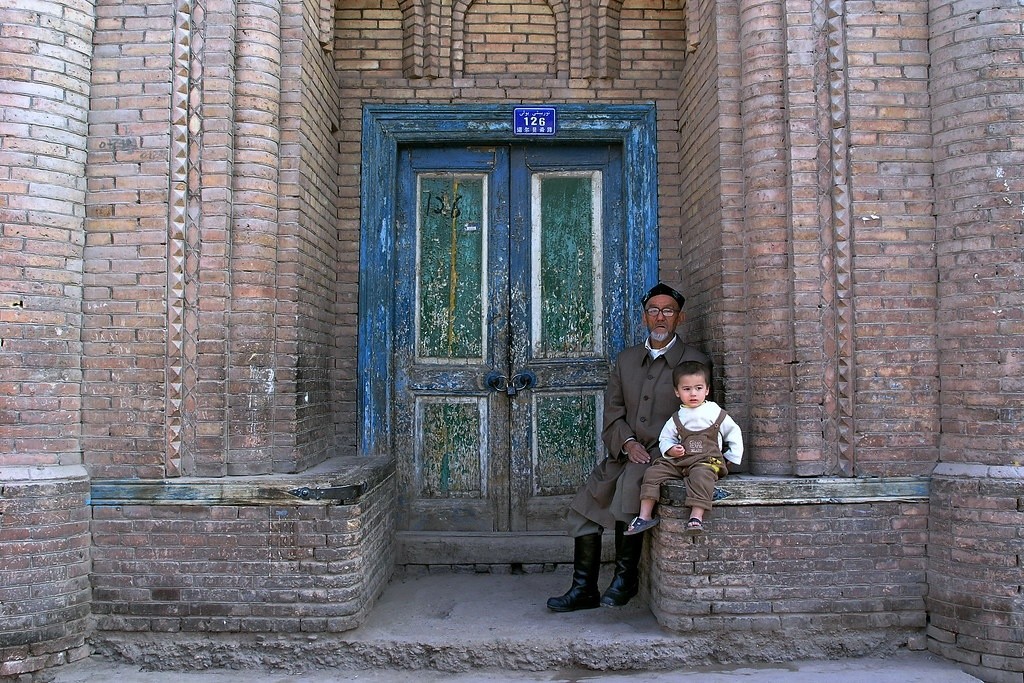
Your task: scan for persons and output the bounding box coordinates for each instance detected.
[623,361,744,535]
[546,285,711,611]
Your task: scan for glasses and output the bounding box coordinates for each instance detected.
[644,307,681,317]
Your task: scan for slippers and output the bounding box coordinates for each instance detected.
[623,514,660,535]
[686,517,703,534]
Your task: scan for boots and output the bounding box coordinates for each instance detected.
[547,533,602,612]
[599,520,645,608]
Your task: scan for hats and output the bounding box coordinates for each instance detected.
[641,283,685,311]
